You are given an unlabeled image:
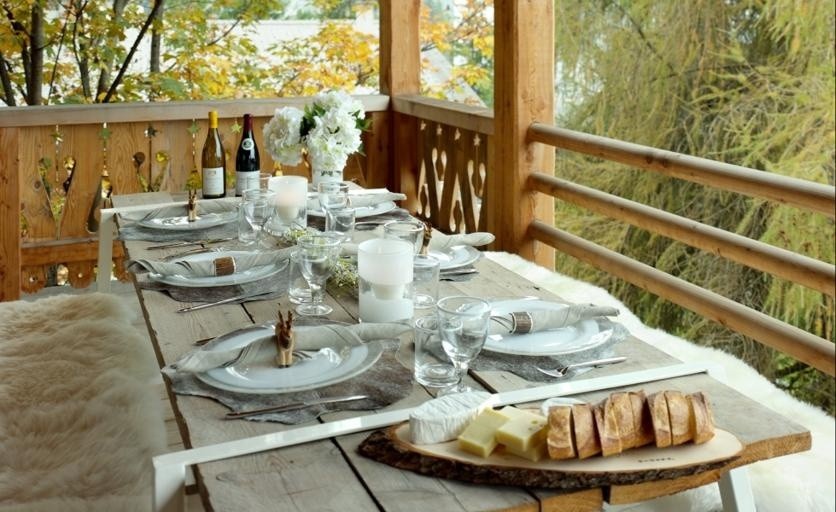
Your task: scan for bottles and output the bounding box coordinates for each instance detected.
[199,111,227,201]
[234,113,262,200]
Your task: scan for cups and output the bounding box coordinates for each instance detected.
[413,253,442,309]
[237,173,356,244]
[384,221,425,255]
[288,251,330,305]
[357,238,415,326]
[414,316,460,388]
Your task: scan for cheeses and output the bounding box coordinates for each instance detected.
[496,410,548,451]
[457,408,509,457]
[497,405,520,420]
[504,440,548,463]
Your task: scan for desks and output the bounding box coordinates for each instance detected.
[110,180,812,511]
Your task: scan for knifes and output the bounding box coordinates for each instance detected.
[223,392,372,420]
[177,286,275,313]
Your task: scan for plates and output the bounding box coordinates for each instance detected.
[147,248,289,288]
[136,214,226,231]
[430,244,482,271]
[307,193,396,219]
[194,318,384,392]
[482,298,613,356]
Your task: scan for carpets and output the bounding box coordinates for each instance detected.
[1,293,170,512]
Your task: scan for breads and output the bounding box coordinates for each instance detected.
[546,391,715,459]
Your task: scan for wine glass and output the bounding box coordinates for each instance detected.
[295,235,338,316]
[437,296,491,395]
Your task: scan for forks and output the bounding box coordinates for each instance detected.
[531,356,628,381]
